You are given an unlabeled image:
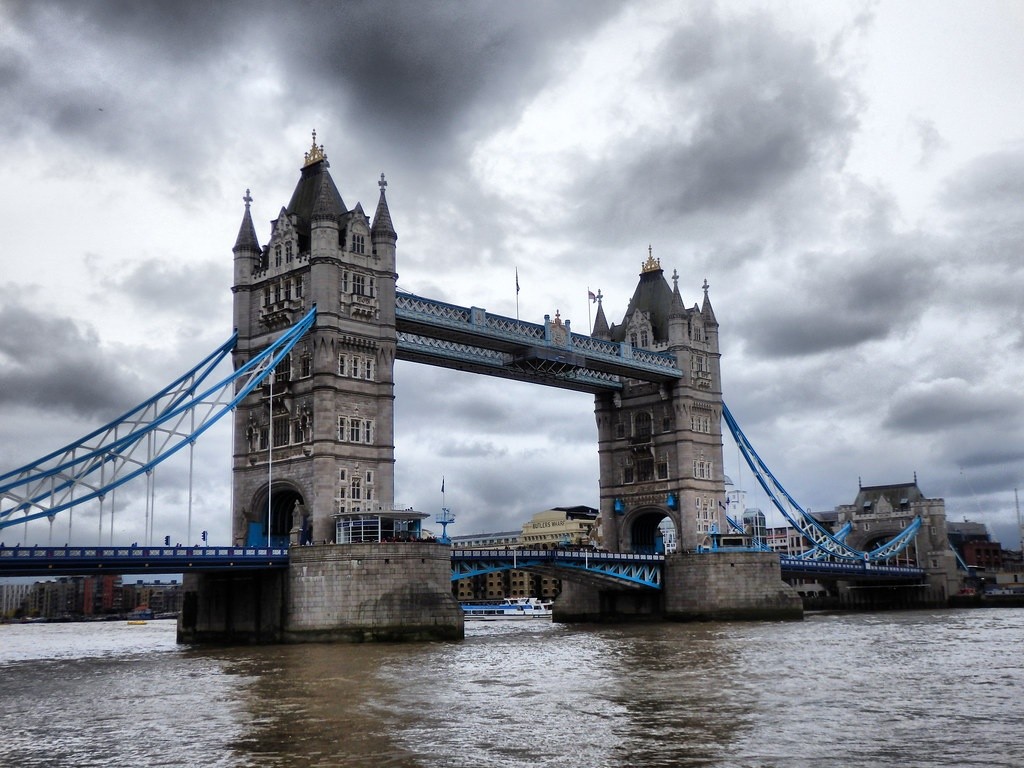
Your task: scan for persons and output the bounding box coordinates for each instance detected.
[323,538,334,544]
[357,534,437,543]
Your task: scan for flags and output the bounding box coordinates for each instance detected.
[516,283,520,294]
[589,291,597,303]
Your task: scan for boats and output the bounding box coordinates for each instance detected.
[127,621,148,625]
[457,596,553,621]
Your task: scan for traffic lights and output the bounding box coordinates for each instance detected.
[202,530,207,541]
[164,536,170,545]
